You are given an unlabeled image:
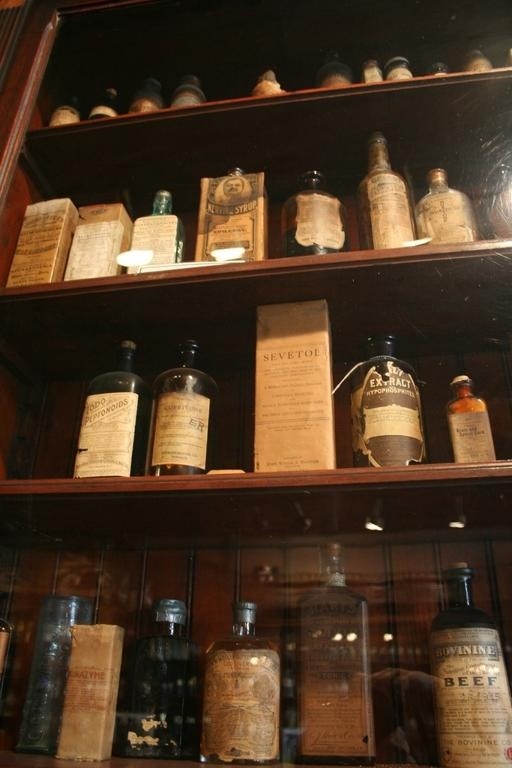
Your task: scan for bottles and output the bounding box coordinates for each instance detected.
[345,330,429,468]
[291,541,376,765]
[280,169,349,258]
[443,373,497,464]
[44,47,511,129]
[353,134,417,248]
[117,598,201,760]
[196,165,269,264]
[125,187,183,275]
[71,337,146,480]
[144,338,221,478]
[422,560,511,768]
[199,598,281,765]
[414,168,480,246]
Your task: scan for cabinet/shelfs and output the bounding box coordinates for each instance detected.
[11,60,512,497]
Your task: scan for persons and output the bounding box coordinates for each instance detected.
[224,177,244,196]
